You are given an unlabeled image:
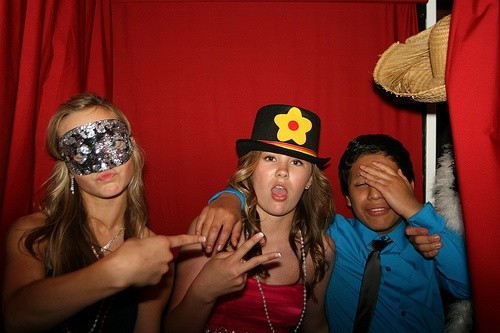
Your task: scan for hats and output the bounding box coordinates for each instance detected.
[236,104,331,170]
[374,11,447,102]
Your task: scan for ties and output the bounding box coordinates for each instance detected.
[353,239,392,333]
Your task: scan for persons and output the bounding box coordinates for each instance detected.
[0,93,208,333]
[152,103,335,333]
[191,134,473,333]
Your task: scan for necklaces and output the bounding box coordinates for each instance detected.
[245,207,309,333]
[83,301,105,333]
[65,205,129,257]
